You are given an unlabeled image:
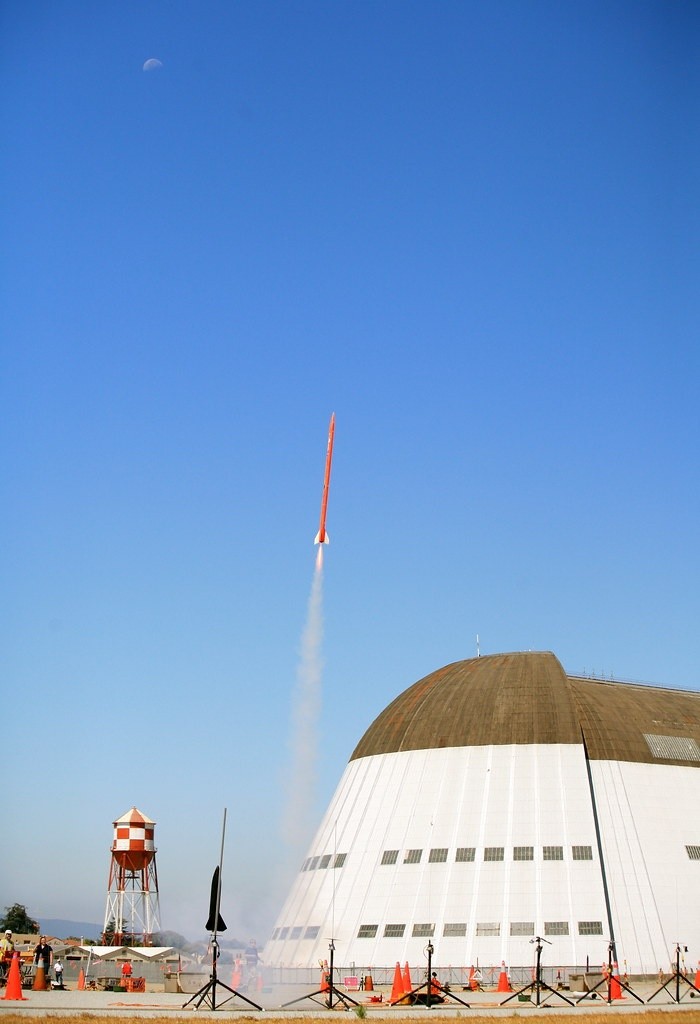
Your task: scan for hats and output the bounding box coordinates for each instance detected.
[5,930,12,935]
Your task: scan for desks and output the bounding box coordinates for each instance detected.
[95,976,120,990]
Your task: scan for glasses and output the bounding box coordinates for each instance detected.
[7,934,11,935]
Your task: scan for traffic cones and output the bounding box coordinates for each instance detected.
[383,962,405,1005]
[363,966,375,992]
[255,973,266,996]
[494,961,512,993]
[1,952,30,1002]
[317,960,333,994]
[601,963,629,1001]
[402,960,415,995]
[30,956,51,993]
[691,961,700,992]
[464,965,481,992]
[74,967,87,992]
[229,958,246,991]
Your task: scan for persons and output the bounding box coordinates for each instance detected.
[121,960,133,978]
[245,939,265,985]
[33,937,53,975]
[0,930,14,977]
[54,959,63,985]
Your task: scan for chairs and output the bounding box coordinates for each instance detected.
[344,975,361,991]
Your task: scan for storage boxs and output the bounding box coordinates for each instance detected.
[121,978,145,992]
[569,974,589,992]
[19,951,33,960]
[163,971,183,993]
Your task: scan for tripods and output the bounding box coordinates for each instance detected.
[181,809,265,1011]
[390,939,471,1011]
[500,936,575,1008]
[278,820,361,1012]
[646,943,700,1003]
[576,942,645,1004]
